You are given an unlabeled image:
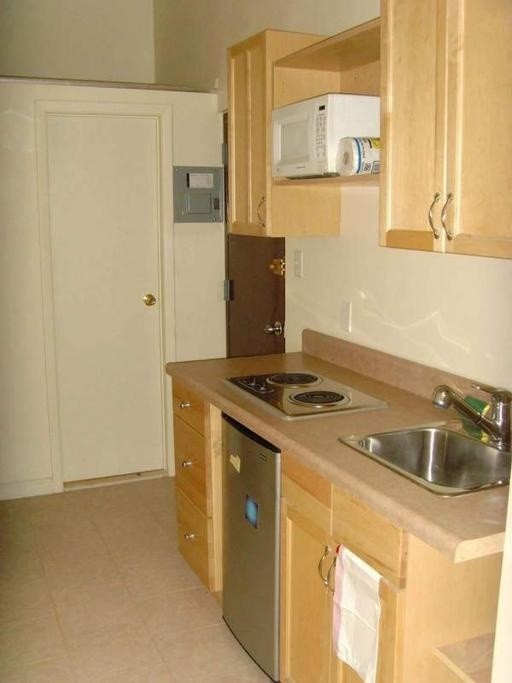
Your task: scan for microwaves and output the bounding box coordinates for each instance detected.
[270,92,382,178]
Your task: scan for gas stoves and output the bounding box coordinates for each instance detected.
[222,368,389,418]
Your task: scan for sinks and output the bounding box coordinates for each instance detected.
[339,424,512,498]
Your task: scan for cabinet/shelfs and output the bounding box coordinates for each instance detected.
[169,370,224,594]
[278,447,504,682]
[375,0,511,257]
[219,26,344,242]
[268,15,381,190]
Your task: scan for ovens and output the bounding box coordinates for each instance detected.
[223,411,279,681]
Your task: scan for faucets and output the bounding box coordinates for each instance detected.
[432,383,511,447]
